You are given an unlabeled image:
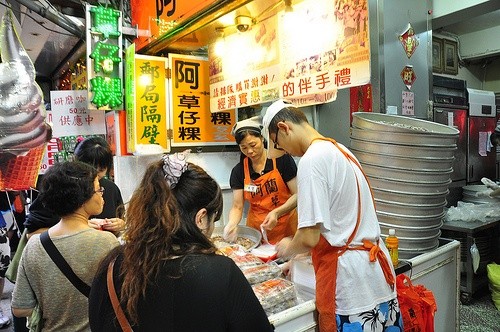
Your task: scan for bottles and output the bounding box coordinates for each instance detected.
[385,228,399,268]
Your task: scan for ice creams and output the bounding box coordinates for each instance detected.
[0,9,52,212]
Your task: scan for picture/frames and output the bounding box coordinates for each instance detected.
[433,35,458,75]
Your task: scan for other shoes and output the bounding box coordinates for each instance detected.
[0,306,10,329]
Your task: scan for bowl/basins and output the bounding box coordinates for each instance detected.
[251,244,279,262]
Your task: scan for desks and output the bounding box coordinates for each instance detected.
[442,222,500,304]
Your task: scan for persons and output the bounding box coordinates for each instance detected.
[10,162,124,332]
[88,156,276,332]
[24,136,125,244]
[223,116,298,245]
[487,130,500,199]
[262,100,405,332]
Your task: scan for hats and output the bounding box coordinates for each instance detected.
[262,98,298,159]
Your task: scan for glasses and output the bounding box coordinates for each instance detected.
[274,118,286,151]
[94,186,105,195]
